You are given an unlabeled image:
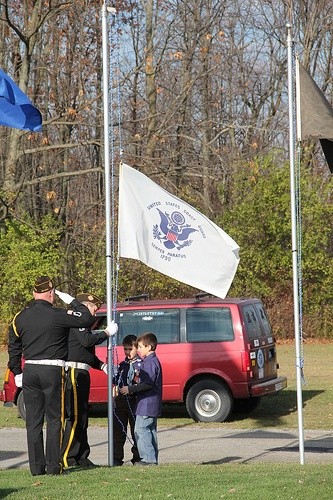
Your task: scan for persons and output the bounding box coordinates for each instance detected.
[113,334,142,467]
[120,333,163,465]
[60,294,120,473]
[6,277,93,476]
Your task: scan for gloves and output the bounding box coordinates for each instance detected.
[100,363,120,377]
[54,289,75,305]
[14,372,24,388]
[105,319,118,336]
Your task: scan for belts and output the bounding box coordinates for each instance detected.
[24,360,65,366]
[65,361,90,372]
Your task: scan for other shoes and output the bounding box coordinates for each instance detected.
[134,461,157,466]
[32,471,67,476]
[69,465,100,471]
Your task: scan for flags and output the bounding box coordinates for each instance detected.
[295,59,333,174]
[117,162,241,300]
[0,68,43,132]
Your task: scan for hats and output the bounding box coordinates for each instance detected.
[76,293,104,309]
[33,276,54,293]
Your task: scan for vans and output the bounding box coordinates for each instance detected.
[0,291,288,423]
